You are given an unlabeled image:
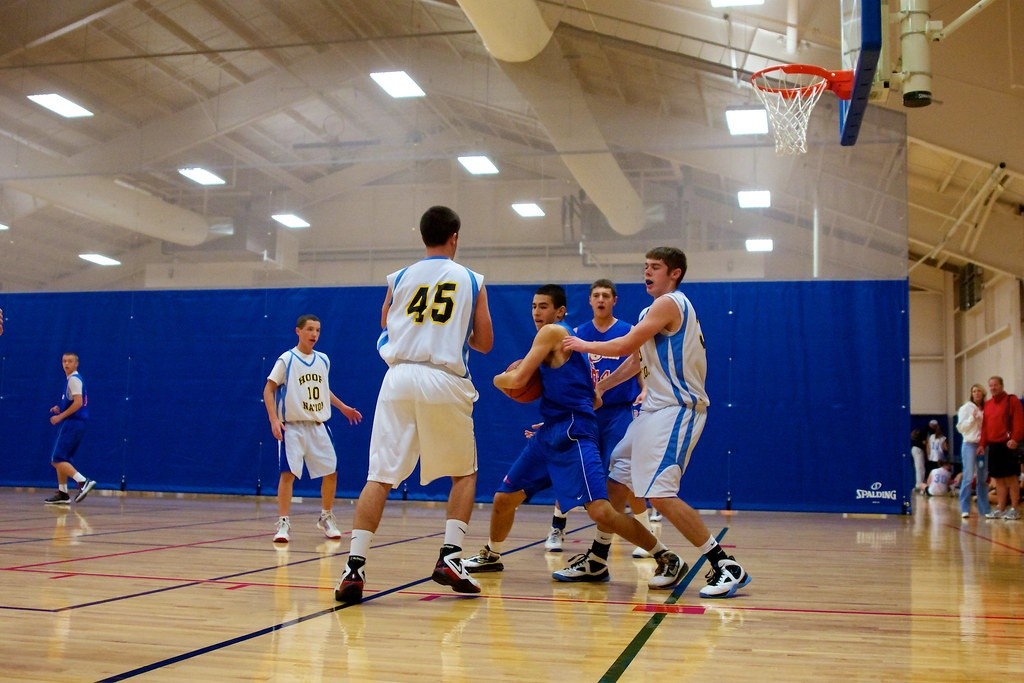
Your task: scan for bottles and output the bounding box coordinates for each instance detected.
[256,479,261,496]
[902,495,907,514]
[121,474,126,491]
[727,492,731,510]
[402,483,407,500]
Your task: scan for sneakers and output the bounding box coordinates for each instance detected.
[631,545,650,559]
[316,513,342,538]
[433,544,482,593]
[544,526,565,552]
[273,517,291,543]
[461,545,504,571]
[650,506,663,522]
[625,506,632,514]
[44,490,71,504]
[74,478,97,503]
[699,556,751,598]
[553,548,611,581]
[648,550,689,589]
[1004,508,1022,520]
[334,556,366,601]
[985,508,1004,519]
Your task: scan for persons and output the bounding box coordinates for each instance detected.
[43,351,97,504]
[545,279,664,559]
[263,314,363,542]
[958,382,994,519]
[977,375,1024,520]
[552,246,752,598]
[459,284,690,589]
[911,419,1024,507]
[335,206,494,603]
[0,309,4,336]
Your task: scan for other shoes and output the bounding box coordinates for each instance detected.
[961,512,969,518]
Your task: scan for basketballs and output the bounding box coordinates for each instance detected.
[503,359,543,403]
